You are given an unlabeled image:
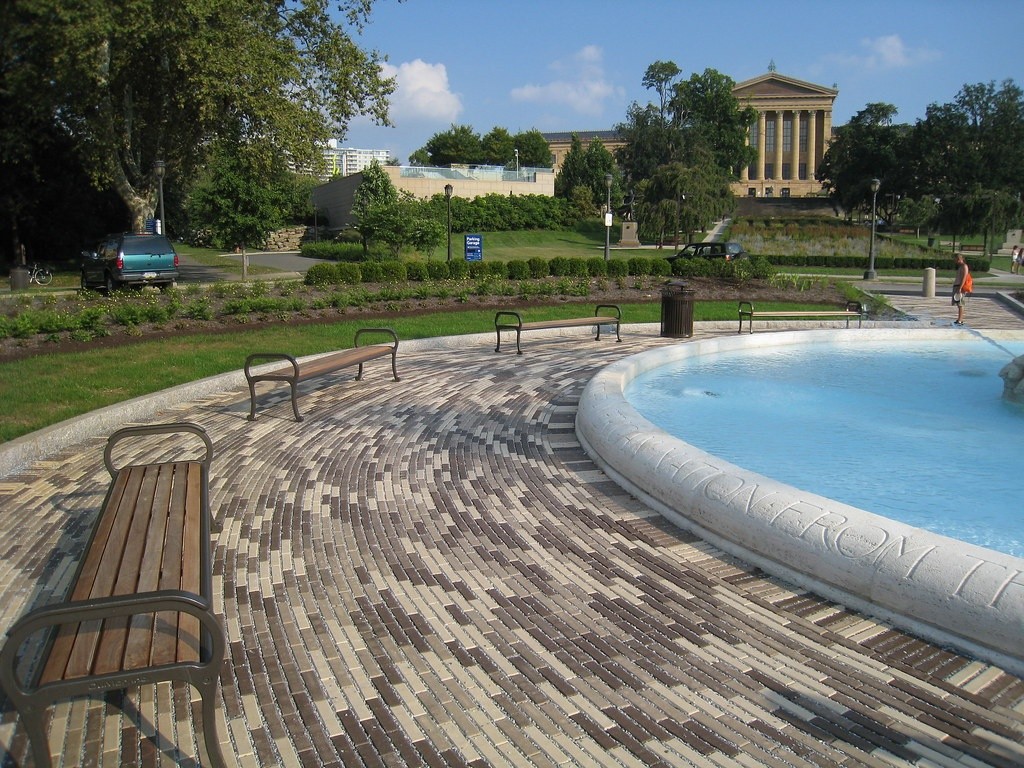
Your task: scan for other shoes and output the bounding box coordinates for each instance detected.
[954,320,963,326]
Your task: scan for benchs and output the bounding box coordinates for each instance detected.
[959,245,986,253]
[737,300,863,333]
[495,305,621,355]
[0,423,227,768]
[244,328,401,422]
[938,240,960,250]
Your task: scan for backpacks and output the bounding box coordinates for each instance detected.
[960,273,972,293]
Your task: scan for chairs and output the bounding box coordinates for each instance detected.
[710,248,718,254]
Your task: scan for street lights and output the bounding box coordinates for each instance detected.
[864,176,881,279]
[514,149,518,180]
[151,158,167,237]
[605,171,612,261]
[444,183,453,261]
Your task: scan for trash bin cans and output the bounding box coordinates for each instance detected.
[659,281,700,339]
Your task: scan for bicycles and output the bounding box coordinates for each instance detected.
[16,262,53,285]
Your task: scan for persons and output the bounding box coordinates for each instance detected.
[951,253,969,326]
[1011,245,1024,275]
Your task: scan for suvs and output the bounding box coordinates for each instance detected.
[663,242,750,277]
[81,230,179,296]
